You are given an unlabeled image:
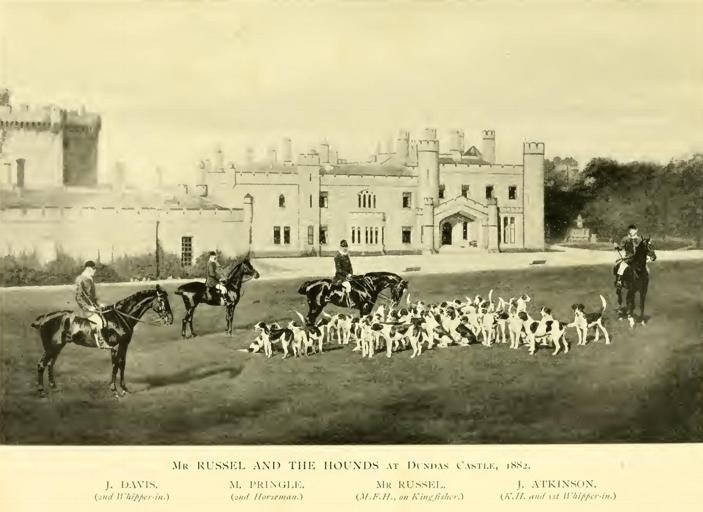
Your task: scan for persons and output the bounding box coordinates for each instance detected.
[205,251,232,307]
[331,238,355,307]
[73,260,107,348]
[615,220,649,296]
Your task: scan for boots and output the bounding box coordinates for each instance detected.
[84,329,96,347]
[616,274,624,289]
[346,292,356,307]
[223,294,232,305]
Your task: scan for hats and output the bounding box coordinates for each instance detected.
[340,240,348,247]
[85,261,95,266]
[628,224,637,229]
[210,251,215,255]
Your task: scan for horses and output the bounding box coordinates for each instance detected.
[25,285,176,397]
[297,270,410,326]
[612,234,658,328]
[175,251,260,339]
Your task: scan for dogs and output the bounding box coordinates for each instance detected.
[236,289,611,358]
